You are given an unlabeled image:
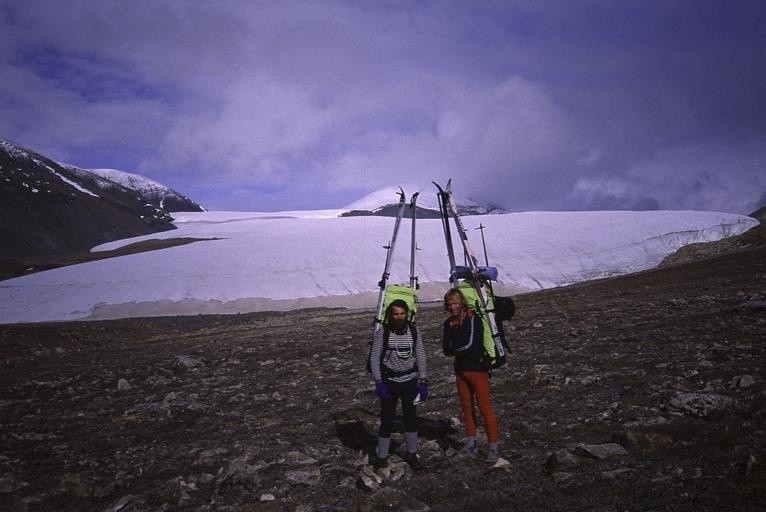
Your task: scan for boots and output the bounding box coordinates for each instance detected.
[485,443,498,464]
[465,436,478,459]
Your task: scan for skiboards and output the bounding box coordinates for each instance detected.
[368,185,421,371]
[432,179,506,358]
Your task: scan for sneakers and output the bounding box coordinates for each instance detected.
[374,457,390,471]
[405,453,423,470]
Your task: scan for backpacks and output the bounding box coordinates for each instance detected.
[368,284,417,375]
[450,280,507,372]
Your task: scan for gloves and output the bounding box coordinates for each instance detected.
[375,380,392,400]
[417,382,428,401]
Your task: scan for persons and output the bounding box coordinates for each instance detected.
[368,298,429,472]
[440,287,504,464]
